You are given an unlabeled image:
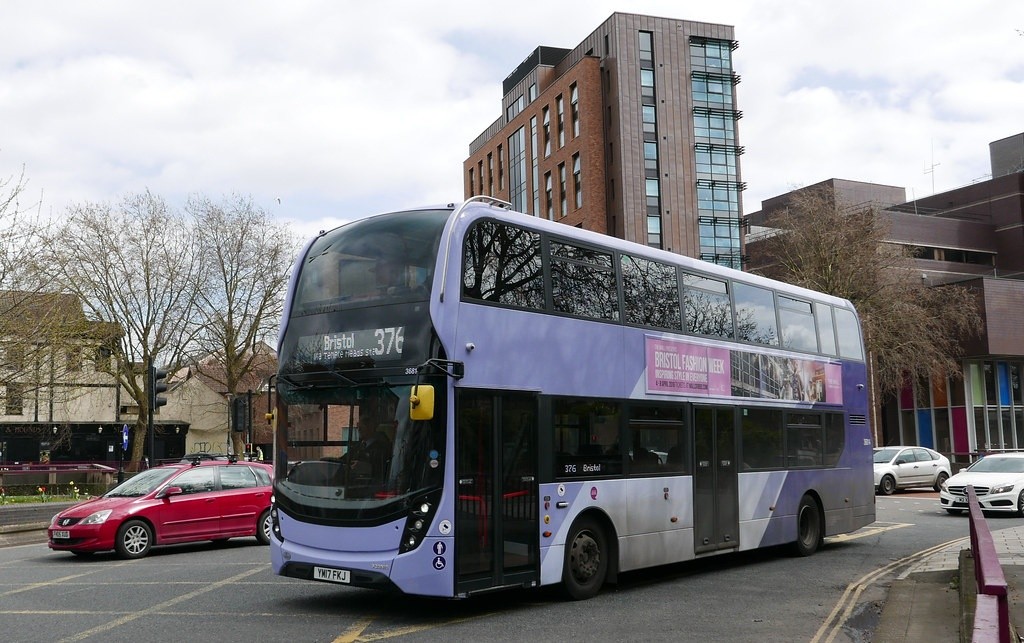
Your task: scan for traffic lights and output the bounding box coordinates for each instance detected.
[245,443,252,455]
[153,366,168,415]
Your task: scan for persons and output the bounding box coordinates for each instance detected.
[256,446,264,462]
[333,411,393,485]
[742,354,826,403]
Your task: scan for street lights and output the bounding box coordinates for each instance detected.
[224,392,234,458]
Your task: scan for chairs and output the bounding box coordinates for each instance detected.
[375,423,398,449]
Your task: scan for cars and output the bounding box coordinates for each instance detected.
[872,446,953,495]
[180,452,227,466]
[63,463,127,483]
[940,451,1024,517]
[46,457,274,562]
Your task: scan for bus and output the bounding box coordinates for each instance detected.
[264,193,878,602]
